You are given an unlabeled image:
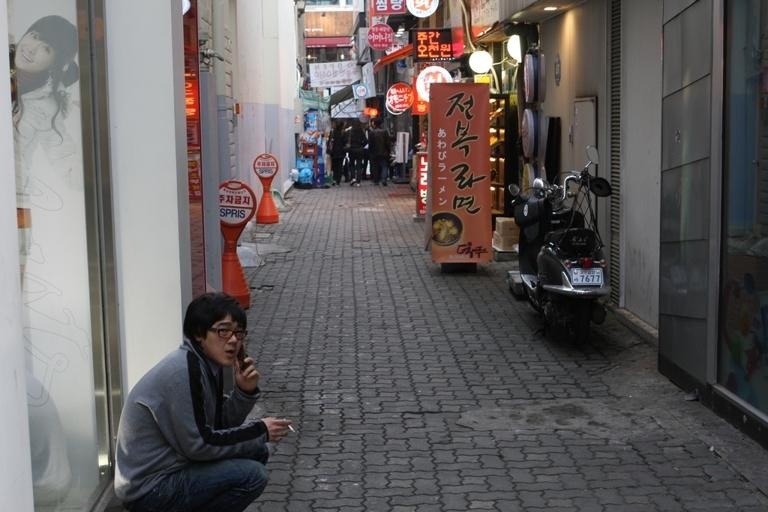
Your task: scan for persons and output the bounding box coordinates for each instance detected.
[325,114,397,188]
[113,290,293,511]
[10,13,82,492]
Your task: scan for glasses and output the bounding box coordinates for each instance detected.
[208,328,248,341]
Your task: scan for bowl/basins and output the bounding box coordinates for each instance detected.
[432,212,463,246]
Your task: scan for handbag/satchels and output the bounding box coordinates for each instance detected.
[344,144,351,152]
[326,141,335,154]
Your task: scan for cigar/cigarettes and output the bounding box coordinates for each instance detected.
[282,417,296,434]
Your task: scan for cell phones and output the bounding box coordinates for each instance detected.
[239,344,249,372]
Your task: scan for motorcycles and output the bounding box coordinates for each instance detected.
[503,141,615,338]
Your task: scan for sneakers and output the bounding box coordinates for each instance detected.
[350,178,360,188]
